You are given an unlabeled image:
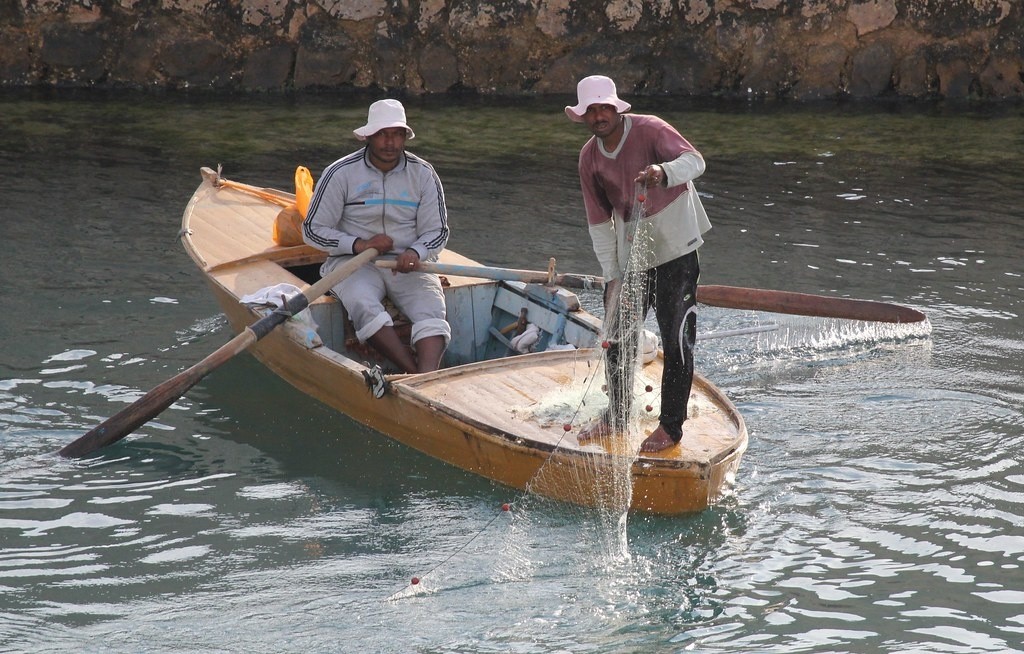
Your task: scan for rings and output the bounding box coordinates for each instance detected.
[409,262,415,266]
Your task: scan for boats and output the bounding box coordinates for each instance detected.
[178,168,749,516]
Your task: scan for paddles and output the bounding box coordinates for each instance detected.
[371,261,928,326]
[56,245,383,457]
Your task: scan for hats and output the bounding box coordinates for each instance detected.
[353,99,415,139]
[565,75,632,122]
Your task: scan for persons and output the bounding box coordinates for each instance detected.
[565,75,713,454]
[301,98,453,375]
